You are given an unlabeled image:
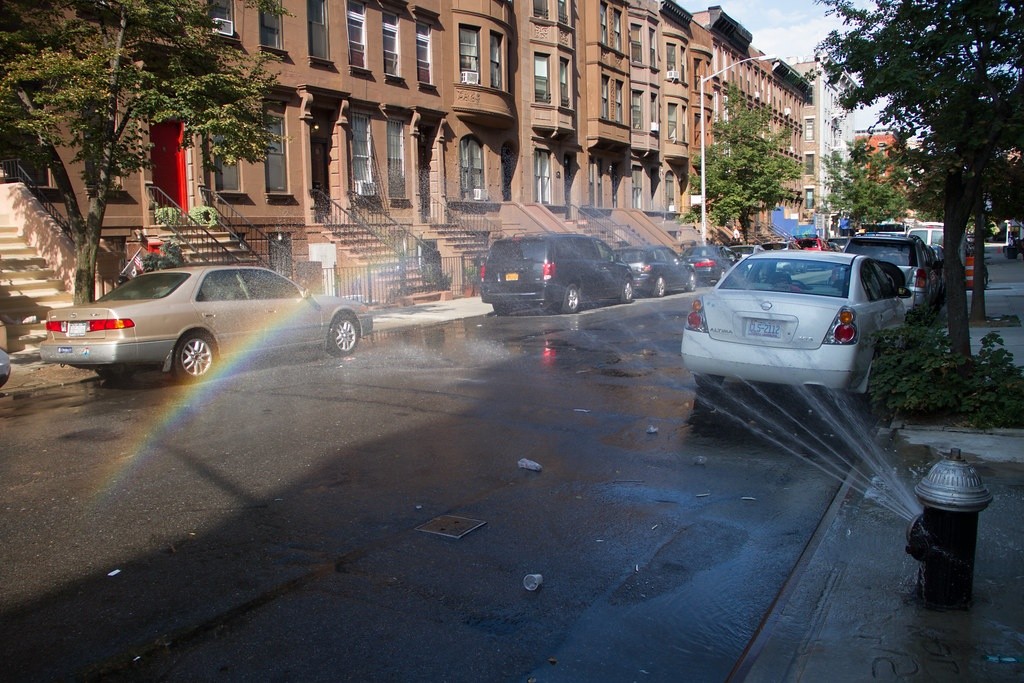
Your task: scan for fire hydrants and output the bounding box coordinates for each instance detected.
[903,447,994,608]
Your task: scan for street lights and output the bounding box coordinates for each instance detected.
[699,54,777,245]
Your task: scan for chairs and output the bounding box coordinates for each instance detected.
[765,272,792,291]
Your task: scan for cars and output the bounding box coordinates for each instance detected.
[729,245,764,265]
[829,235,852,251]
[613,244,697,298]
[679,246,735,282]
[762,241,798,250]
[39,263,374,382]
[681,248,913,401]
[793,237,830,253]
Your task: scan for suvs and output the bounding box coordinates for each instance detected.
[841,230,940,327]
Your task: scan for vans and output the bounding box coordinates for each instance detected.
[479,232,637,316]
[906,226,946,248]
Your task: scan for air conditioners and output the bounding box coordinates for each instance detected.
[355,179,376,196]
[212,17,233,37]
[651,122,660,132]
[669,205,677,212]
[667,71,679,79]
[473,189,489,201]
[461,71,479,84]
